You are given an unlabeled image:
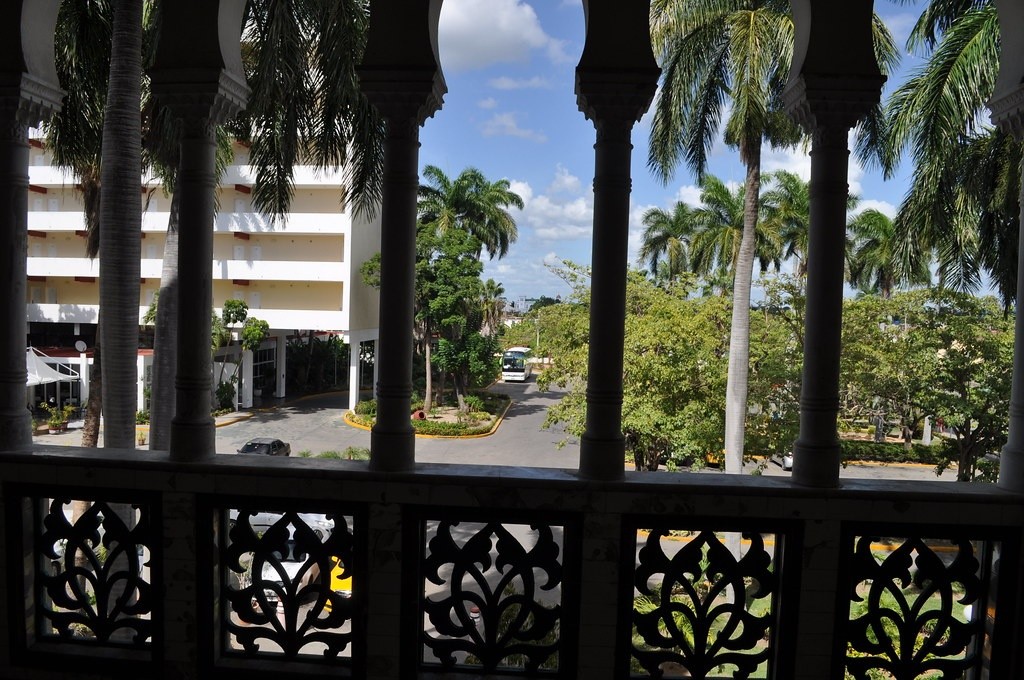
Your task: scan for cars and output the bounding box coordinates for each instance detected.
[229,511,356,614]
[771,451,793,469]
[656,439,725,465]
[237,438,292,457]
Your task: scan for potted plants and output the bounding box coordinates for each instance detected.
[32,419,40,435]
[137,431,146,445]
[40,402,76,434]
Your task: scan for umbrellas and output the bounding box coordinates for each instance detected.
[26,346,79,387]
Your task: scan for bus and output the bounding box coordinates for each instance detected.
[499,347,532,382]
